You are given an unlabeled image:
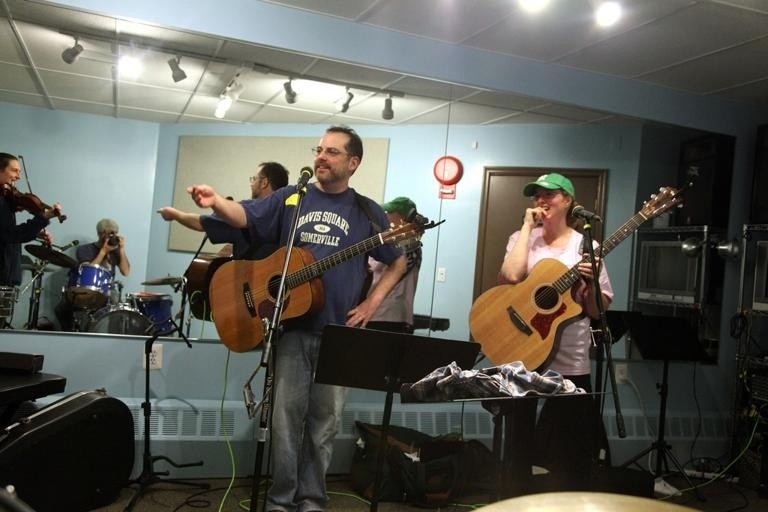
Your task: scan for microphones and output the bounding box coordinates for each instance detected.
[295,167,314,194]
[571,204,602,221]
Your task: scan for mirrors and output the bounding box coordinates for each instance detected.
[0,0,737,364]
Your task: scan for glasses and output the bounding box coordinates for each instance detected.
[248,176,265,183]
[311,146,348,158]
[528,189,561,199]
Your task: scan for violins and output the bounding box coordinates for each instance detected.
[10,186,66,223]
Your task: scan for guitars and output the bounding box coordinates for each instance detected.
[209,222,426,351]
[469,186,685,376]
[187,244,232,320]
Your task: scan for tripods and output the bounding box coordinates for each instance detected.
[620,361,707,504]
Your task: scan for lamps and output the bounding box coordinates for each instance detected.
[60,29,405,121]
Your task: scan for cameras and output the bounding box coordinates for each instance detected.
[108,231,119,246]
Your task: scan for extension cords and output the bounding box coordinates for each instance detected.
[684,469,740,484]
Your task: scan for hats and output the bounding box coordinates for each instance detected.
[380,196,416,217]
[522,172,575,200]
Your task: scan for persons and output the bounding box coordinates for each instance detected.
[0,152,64,328]
[497,172,618,489]
[156,161,291,262]
[54,219,131,333]
[366,195,425,334]
[183,122,414,511]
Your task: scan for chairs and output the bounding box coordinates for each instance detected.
[596,308,643,360]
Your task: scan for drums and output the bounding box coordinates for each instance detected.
[128,293,173,336]
[81,304,158,336]
[65,262,111,308]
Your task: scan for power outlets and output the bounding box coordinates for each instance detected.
[143,344,164,368]
[435,268,445,282]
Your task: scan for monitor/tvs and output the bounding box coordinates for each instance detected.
[637,240,698,304]
[752,241,768,312]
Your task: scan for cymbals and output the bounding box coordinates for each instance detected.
[141,279,183,286]
[25,244,79,269]
